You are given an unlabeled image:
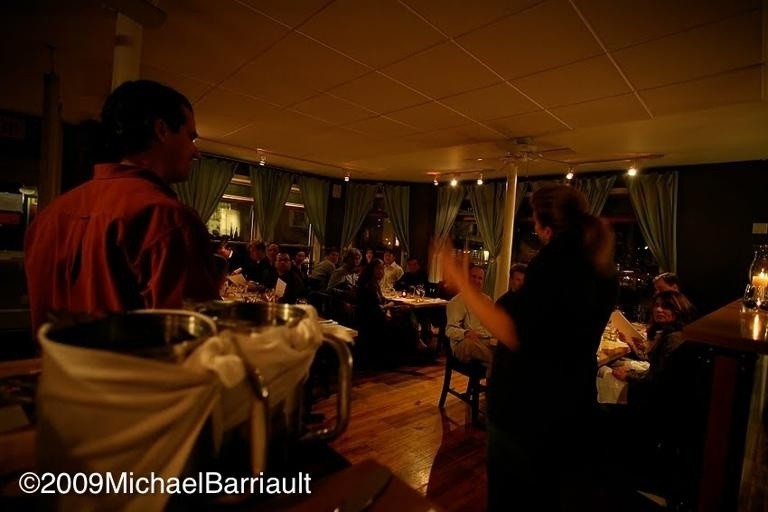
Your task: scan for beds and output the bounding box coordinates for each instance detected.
[681,293,768,470]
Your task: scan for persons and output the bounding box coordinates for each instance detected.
[359,246,380,284]
[312,249,338,288]
[231,240,274,283]
[439,183,618,508]
[377,247,404,288]
[292,251,308,281]
[445,265,498,382]
[259,251,303,304]
[394,257,432,346]
[355,257,439,364]
[612,291,709,511]
[495,262,527,308]
[327,249,362,301]
[266,242,280,267]
[21,80,224,352]
[653,273,678,295]
[217,240,247,274]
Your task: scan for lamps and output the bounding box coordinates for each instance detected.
[432,179,438,186]
[258,155,267,166]
[344,172,350,181]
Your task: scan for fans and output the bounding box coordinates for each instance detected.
[464,142,576,179]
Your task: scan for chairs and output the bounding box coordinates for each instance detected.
[437,306,487,427]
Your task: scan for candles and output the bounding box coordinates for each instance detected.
[752,268,768,288]
